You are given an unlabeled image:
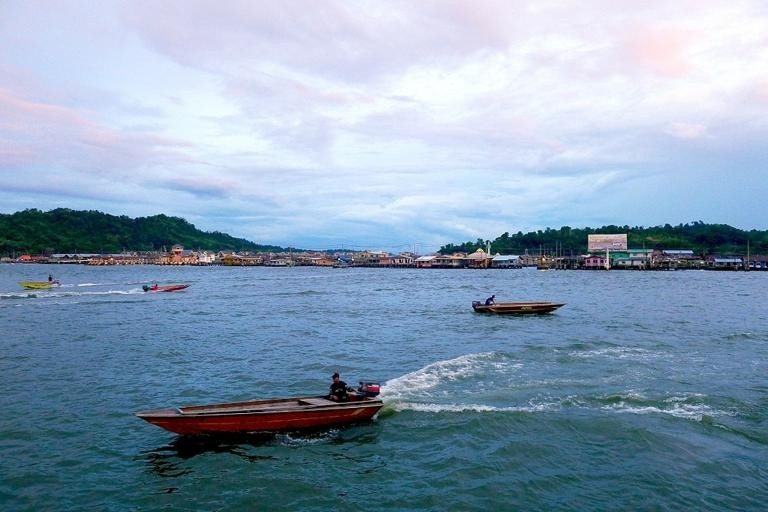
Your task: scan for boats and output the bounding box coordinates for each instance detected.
[472,301,567,314]
[16,280,61,288]
[142,285,190,291]
[135,382,384,436]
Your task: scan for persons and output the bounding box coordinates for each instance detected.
[484,295,497,306]
[329,372,359,402]
[48,274,52,281]
[152,283,158,290]
